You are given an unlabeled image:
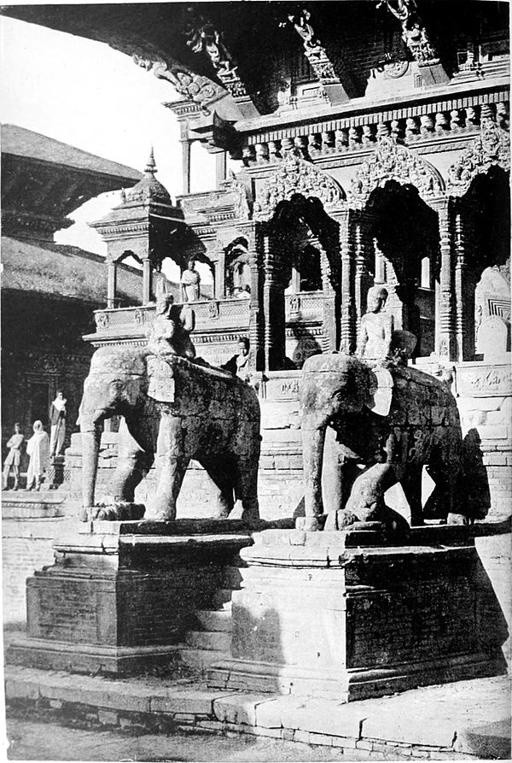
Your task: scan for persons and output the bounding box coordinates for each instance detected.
[220,338,250,382]
[352,285,397,363]
[145,292,175,354]
[47,387,69,457]
[24,419,50,491]
[152,261,168,301]
[2,421,24,491]
[181,258,201,302]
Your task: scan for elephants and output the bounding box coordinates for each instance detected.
[292,353,464,530]
[77,345,262,523]
